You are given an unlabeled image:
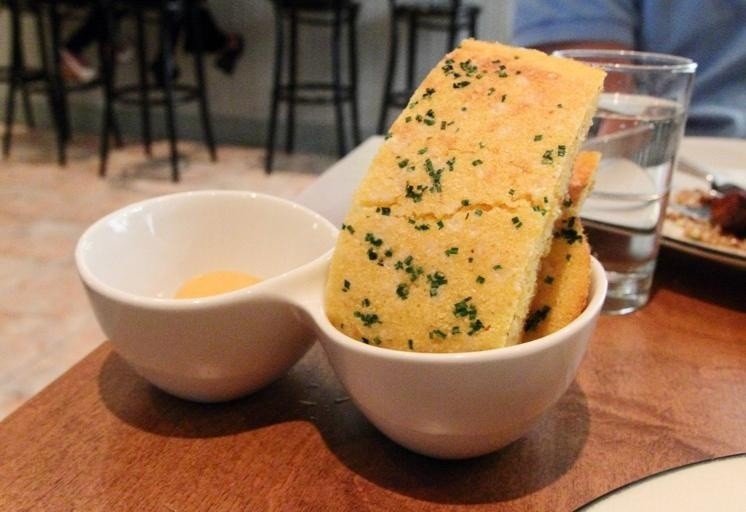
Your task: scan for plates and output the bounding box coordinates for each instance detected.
[580,136,746,268]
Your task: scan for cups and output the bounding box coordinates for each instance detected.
[551,49,697,315]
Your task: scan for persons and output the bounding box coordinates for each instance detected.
[513,1,746,150]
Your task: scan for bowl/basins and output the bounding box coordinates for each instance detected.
[74,188,609,461]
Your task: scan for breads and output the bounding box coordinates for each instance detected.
[326,38,610,353]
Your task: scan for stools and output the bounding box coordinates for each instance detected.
[98,0,216,181]
[265,0,360,174]
[377,2,481,135]
[2,1,121,167]
